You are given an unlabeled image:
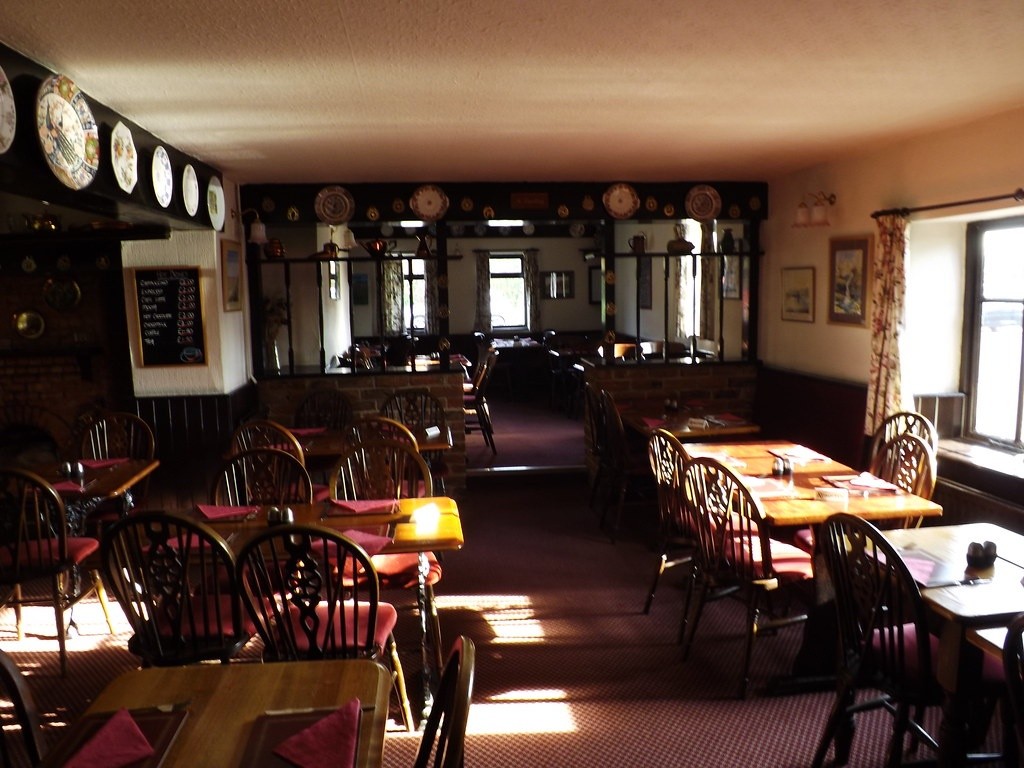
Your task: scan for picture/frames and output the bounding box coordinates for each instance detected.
[826,233,875,330]
[640,256,653,310]
[781,266,816,323]
[589,266,603,305]
[541,269,576,300]
[220,238,243,312]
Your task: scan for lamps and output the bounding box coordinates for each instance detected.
[231,206,271,246]
[790,190,837,228]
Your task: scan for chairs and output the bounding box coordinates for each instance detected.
[796,434,937,657]
[194,448,313,596]
[337,417,426,500]
[811,512,985,768]
[0,467,114,676]
[464,345,495,440]
[639,428,762,636]
[379,388,452,498]
[83,411,155,575]
[868,410,939,529]
[599,389,661,544]
[679,457,820,696]
[230,420,330,503]
[584,381,653,525]
[464,351,502,456]
[101,507,284,670]
[333,438,442,671]
[235,523,415,732]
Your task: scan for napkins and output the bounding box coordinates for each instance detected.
[287,428,325,435]
[451,354,462,361]
[642,416,664,429]
[26,481,81,492]
[142,532,210,551]
[79,459,126,468]
[313,529,393,579]
[63,706,153,768]
[867,551,961,588]
[198,502,257,519]
[715,412,743,422]
[274,697,360,768]
[332,499,399,514]
[452,357,468,365]
[787,444,826,461]
[850,471,899,492]
[383,423,413,432]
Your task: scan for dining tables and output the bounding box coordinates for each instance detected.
[412,636,478,768]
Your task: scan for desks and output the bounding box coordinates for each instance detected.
[406,354,472,383]
[577,354,711,371]
[837,523,1024,768]
[225,427,453,495]
[683,438,944,678]
[491,336,542,399]
[40,659,390,768]
[24,460,160,597]
[141,494,465,673]
[624,411,761,506]
[357,342,392,369]
